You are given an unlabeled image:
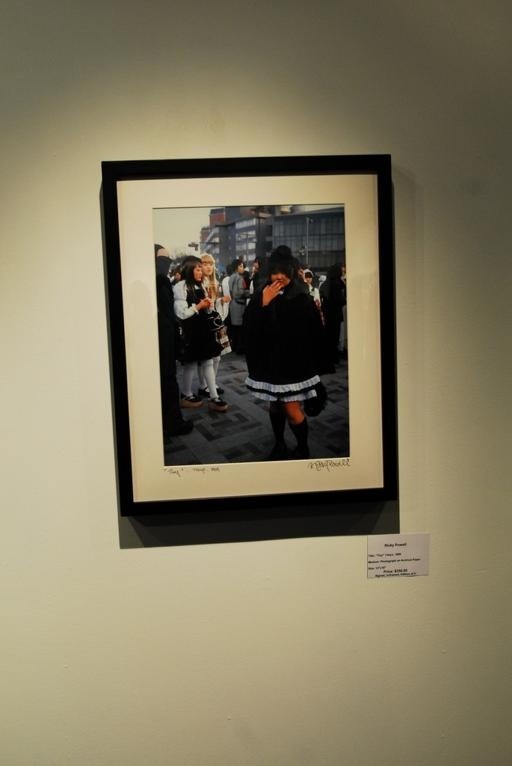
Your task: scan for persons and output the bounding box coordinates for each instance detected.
[154,243,348,461]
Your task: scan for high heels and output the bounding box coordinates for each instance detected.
[180,386,228,411]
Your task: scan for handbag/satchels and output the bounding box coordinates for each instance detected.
[234,295,247,304]
[207,311,224,333]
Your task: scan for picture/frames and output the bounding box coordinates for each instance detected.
[101,153,396,517]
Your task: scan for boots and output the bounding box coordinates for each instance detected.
[268,407,310,460]
